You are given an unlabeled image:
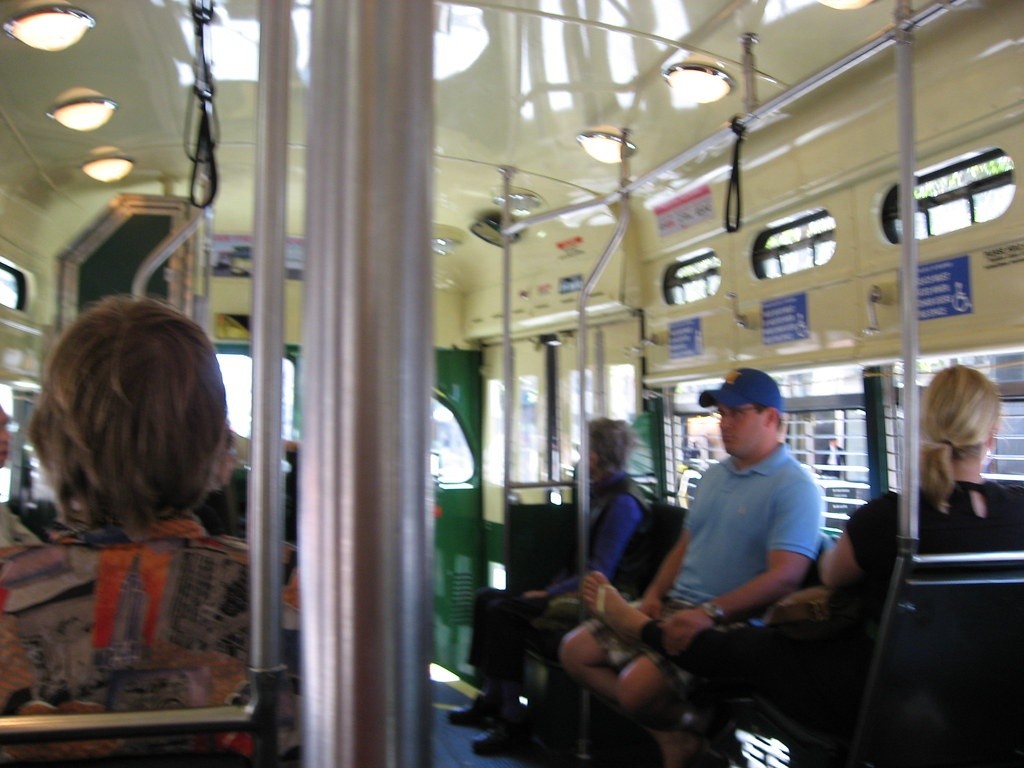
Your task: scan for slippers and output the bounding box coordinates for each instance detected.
[582,570,640,645]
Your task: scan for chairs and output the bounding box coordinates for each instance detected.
[527,503,687,666]
[755,550,1024,768]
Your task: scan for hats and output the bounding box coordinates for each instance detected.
[698,367,786,413]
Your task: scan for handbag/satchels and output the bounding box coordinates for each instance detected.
[767,586,833,624]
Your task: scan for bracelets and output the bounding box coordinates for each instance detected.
[701,602,730,624]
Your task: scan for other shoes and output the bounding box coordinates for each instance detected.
[470,721,542,758]
[447,686,520,729]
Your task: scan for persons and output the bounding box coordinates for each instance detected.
[447,419,654,755]
[558,364,1024,768]
[0,295,302,768]
